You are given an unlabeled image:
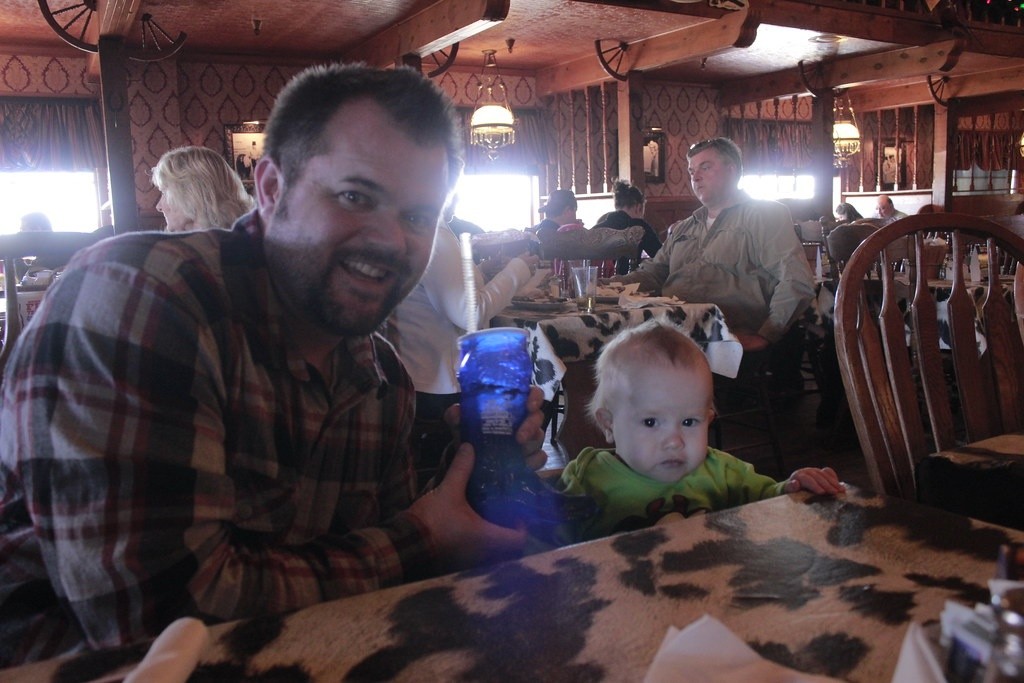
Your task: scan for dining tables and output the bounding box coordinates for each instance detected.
[488,284,744,455]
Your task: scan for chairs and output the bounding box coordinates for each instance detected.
[379,192,1023,529]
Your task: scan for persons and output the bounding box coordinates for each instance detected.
[556,318,846,543]
[21,213,52,231]
[538,189,584,231]
[592,180,665,274]
[396,195,540,490]
[567,136,813,403]
[835,203,863,221]
[874,195,907,219]
[0,60,548,670]
[152,146,256,233]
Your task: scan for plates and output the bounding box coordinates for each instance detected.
[595,296,619,304]
[511,301,565,311]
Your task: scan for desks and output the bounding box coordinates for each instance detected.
[0,482,1023,683]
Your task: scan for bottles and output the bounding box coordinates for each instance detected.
[982,587,1022,681]
[455,325,533,507]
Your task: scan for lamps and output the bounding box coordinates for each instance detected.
[832,88,860,169]
[470,49,517,161]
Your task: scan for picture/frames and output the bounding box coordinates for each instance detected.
[616,128,665,184]
[223,123,267,191]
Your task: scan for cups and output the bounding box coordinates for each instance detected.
[566,259,591,303]
[571,266,598,313]
